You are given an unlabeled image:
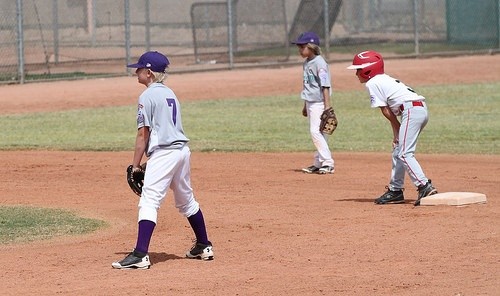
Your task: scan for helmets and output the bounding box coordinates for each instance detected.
[347,49,384,80]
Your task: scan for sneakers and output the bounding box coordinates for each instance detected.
[185,242,214,260]
[318,166,335,174]
[414,179,437,206]
[372,189,405,204]
[111,252,150,270]
[302,165,318,173]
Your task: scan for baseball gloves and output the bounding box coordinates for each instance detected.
[126,163,146,197]
[319,107,337,135]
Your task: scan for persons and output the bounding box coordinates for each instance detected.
[290,32,334,174]
[111,51,214,269]
[345,51,437,206]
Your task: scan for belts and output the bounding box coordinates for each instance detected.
[400,102,423,111]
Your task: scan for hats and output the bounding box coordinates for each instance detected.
[292,33,320,45]
[129,50,168,72]
